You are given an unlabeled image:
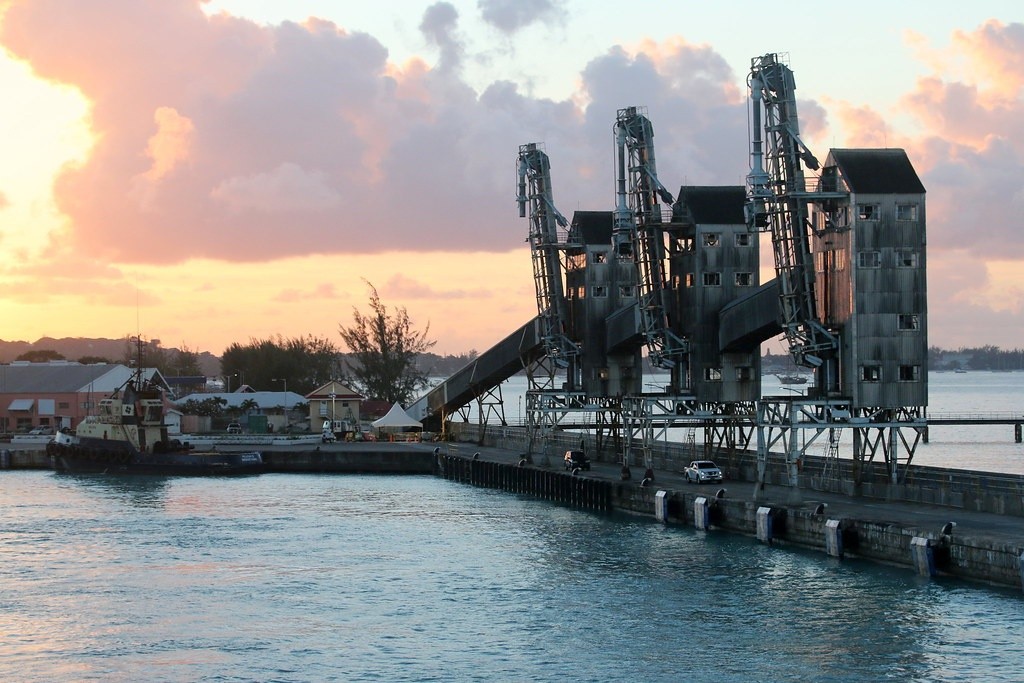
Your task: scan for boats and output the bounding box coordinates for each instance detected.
[955,370,967,373]
[992,369,1012,372]
[44,332,265,478]
[776,375,809,384]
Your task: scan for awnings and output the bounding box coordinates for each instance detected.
[7,399,35,417]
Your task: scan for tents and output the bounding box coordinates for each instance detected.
[370,400,423,444]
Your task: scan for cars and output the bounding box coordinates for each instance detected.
[226,423,242,434]
[563,451,591,471]
[684,461,722,485]
[29,424,54,435]
